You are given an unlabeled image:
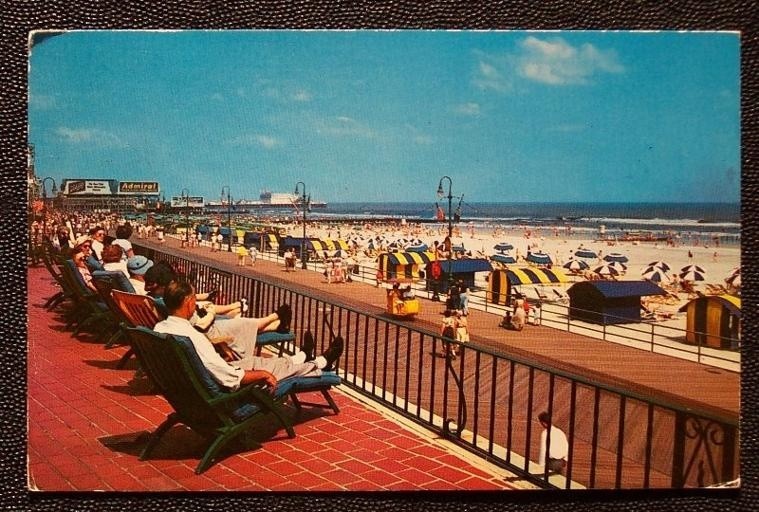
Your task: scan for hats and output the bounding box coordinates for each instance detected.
[74,235,94,247]
[127,255,179,291]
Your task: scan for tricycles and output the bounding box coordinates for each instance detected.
[385,288,418,320]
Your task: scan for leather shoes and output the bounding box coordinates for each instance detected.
[208,288,217,300]
[321,336,343,371]
[301,330,314,363]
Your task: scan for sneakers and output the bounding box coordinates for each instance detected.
[275,304,293,333]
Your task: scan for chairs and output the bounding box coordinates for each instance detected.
[39,236,341,473]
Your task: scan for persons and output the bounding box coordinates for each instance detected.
[28,202,742,398]
[538,410,570,473]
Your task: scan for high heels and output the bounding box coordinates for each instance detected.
[237,298,248,314]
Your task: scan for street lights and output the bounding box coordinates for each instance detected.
[294,182,307,268]
[222,186,232,251]
[42,177,58,233]
[158,190,166,234]
[436,177,453,280]
[182,188,189,243]
